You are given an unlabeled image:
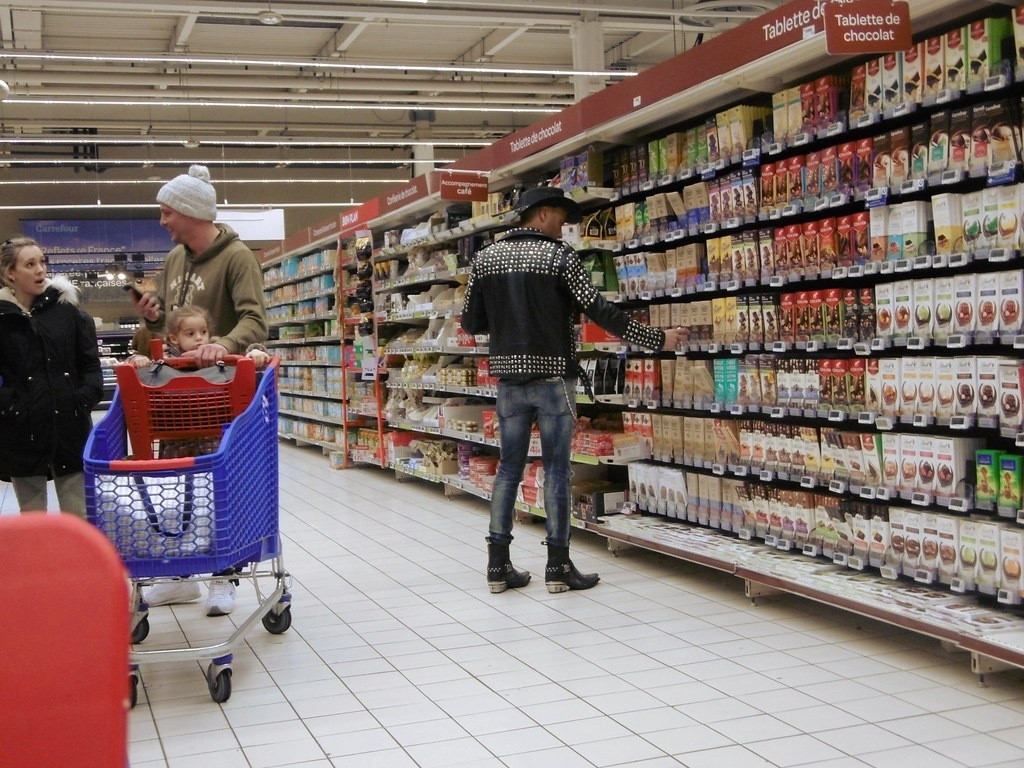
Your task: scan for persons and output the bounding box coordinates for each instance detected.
[126,163,270,616]
[0,232,103,524]
[457,186,692,594]
[127,304,270,460]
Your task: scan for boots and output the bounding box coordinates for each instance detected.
[485,536,531,593]
[541,541,600,594]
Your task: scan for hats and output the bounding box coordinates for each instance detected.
[509,187,582,224]
[155,164,217,222]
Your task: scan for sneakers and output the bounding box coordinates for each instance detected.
[141,574,202,607]
[205,575,235,615]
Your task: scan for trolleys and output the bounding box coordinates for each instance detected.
[82,338,293,710]
